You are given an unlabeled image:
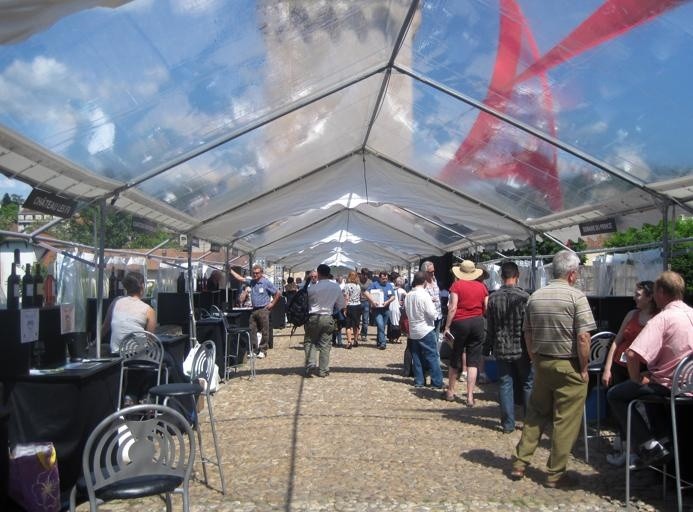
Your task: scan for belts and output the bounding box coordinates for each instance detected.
[253,306,265,310]
[539,353,572,359]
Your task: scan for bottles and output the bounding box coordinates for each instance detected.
[176,272,185,293]
[44,272,56,307]
[31,264,43,307]
[21,263,33,308]
[108,269,123,298]
[6,263,19,309]
[196,275,208,292]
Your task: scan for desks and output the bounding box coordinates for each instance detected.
[182,306,254,379]
[11,333,189,506]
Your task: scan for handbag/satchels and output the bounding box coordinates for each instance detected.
[7,440,61,511]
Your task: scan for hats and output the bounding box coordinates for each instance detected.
[451,260,484,282]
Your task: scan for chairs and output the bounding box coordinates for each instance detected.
[624,350,692,511]
[579,329,622,462]
[214,306,255,384]
[69,331,226,511]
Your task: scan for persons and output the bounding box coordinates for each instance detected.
[599,278,662,468]
[507,248,600,490]
[91,270,159,411]
[484,261,532,436]
[606,267,692,476]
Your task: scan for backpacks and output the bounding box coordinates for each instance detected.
[286,277,309,328]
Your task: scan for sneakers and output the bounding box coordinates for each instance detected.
[333,335,386,350]
[511,467,524,477]
[305,364,330,377]
[606,437,673,468]
[248,350,256,358]
[548,475,579,488]
[257,351,266,358]
